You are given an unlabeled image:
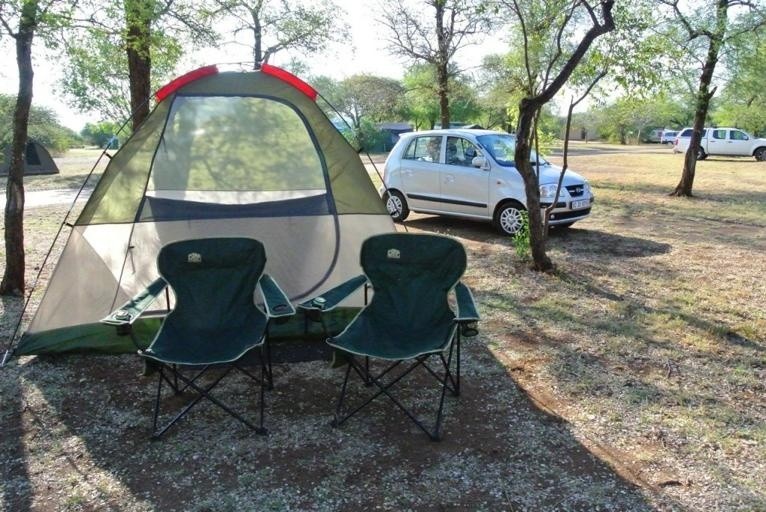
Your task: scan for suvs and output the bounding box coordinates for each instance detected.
[380,128,595,237]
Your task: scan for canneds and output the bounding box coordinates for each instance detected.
[115,310,130,336]
[311,297,327,321]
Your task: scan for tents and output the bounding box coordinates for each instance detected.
[0,62,408,369]
[1,133,59,176]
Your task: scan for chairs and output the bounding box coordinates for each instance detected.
[294,228,481,444]
[95,236,295,448]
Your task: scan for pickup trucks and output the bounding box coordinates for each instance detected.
[674,128,766,162]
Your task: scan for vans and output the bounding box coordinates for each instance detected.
[640,127,663,143]
[661,129,681,146]
